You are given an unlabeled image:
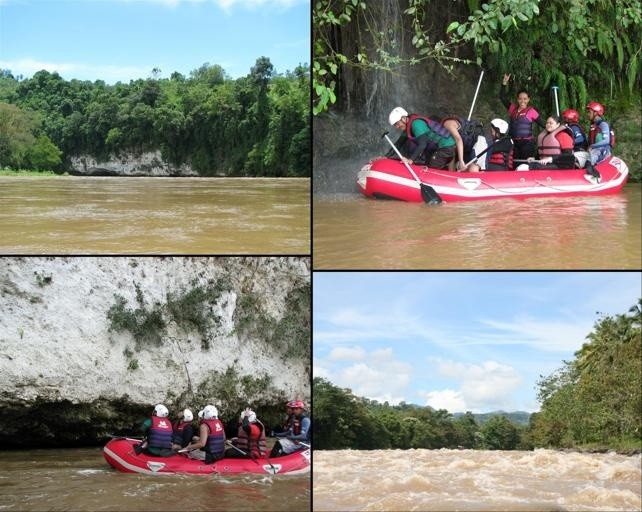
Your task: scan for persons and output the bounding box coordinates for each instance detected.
[284,401,292,430]
[572,102,612,183]
[485,118,515,171]
[563,109,587,151]
[500,73,547,171]
[516,116,574,171]
[140,404,266,464]
[430,112,488,173]
[382,106,456,171]
[268,400,311,458]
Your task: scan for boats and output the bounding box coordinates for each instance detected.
[355,153,628,204]
[102,433,310,481]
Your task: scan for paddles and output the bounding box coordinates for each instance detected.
[228,443,259,465]
[381,132,442,204]
[514,154,575,169]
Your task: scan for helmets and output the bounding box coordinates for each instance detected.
[198,405,218,420]
[587,102,604,116]
[491,119,509,137]
[388,107,409,126]
[287,400,304,408]
[152,404,169,418]
[562,110,579,123]
[182,409,193,422]
[240,410,257,423]
